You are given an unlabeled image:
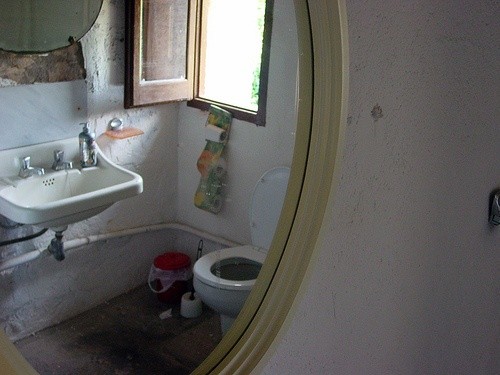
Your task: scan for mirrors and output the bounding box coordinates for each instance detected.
[0,0,347,375]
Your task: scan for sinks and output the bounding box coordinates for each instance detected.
[0,135,144,228]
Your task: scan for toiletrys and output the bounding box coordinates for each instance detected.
[78,122,97,168]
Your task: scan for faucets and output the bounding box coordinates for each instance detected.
[52,149,73,170]
[18,156,44,178]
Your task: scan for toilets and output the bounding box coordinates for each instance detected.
[192,165,291,340]
